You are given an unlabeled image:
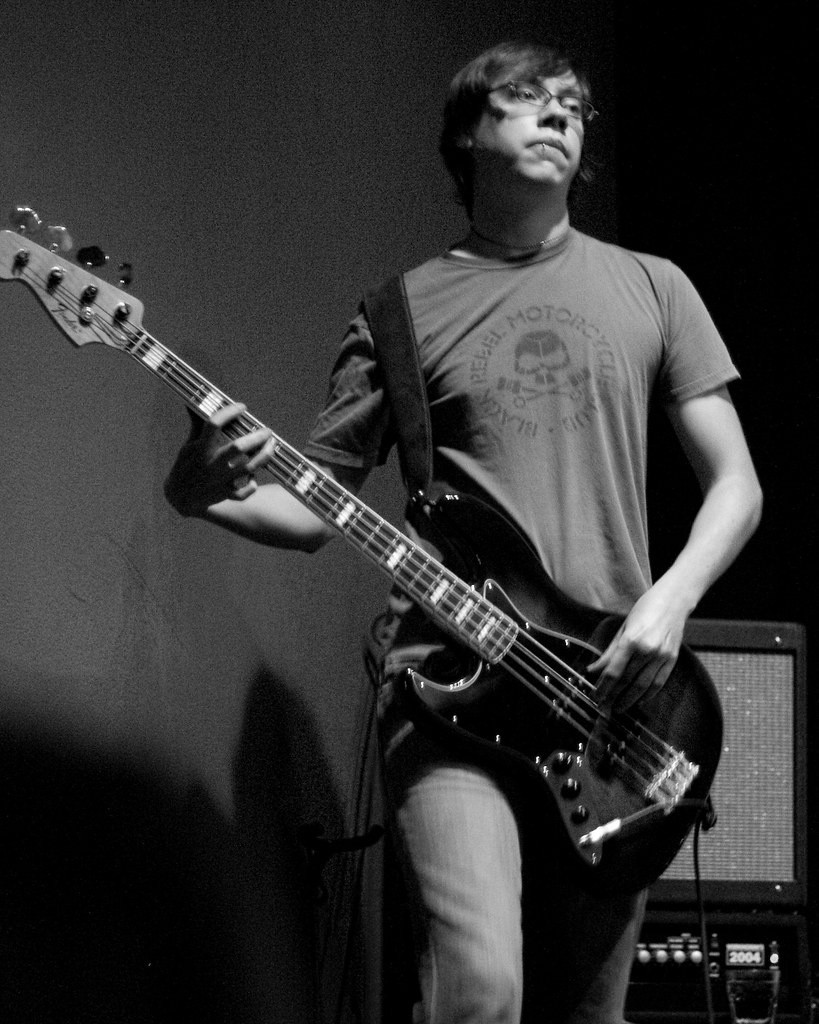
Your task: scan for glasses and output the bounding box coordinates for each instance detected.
[483,81,599,122]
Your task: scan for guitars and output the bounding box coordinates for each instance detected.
[0,205,726,898]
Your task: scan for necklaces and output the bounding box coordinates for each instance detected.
[469,226,566,248]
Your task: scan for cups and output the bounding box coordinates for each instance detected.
[726,969,780,1024]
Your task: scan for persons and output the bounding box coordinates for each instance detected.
[163,42,763,1024]
